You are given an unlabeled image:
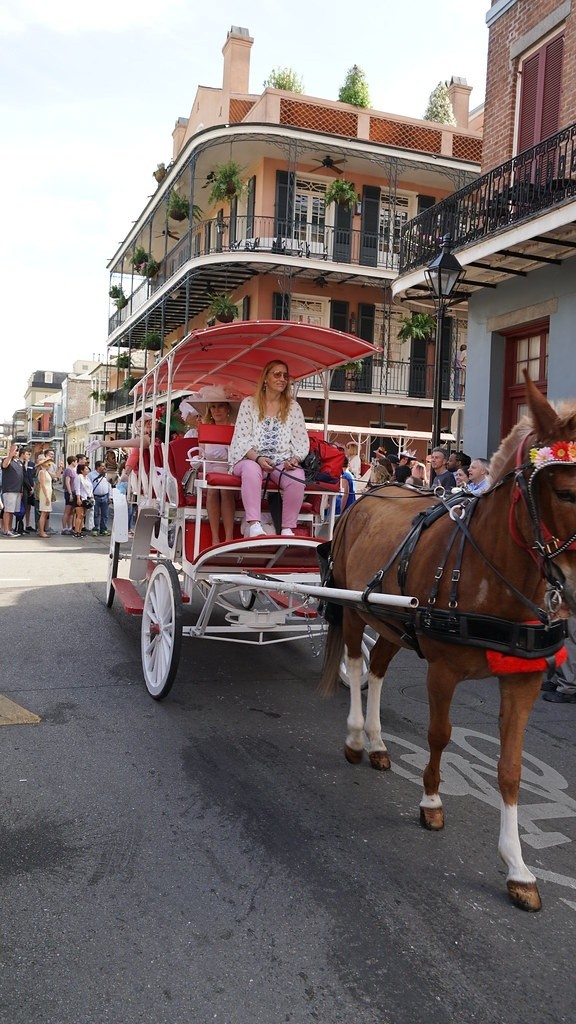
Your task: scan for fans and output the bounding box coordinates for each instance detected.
[155,229,180,241]
[203,170,216,189]
[308,155,348,175]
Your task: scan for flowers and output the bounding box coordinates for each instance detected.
[529,440,576,467]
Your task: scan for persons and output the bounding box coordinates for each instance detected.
[374,447,490,494]
[119,463,135,533]
[227,359,309,536]
[85,413,160,452]
[184,415,204,438]
[43,449,59,535]
[199,402,238,545]
[0,443,37,538]
[76,454,111,536]
[327,441,361,516]
[458,344,467,401]
[34,454,62,538]
[72,464,94,539]
[61,456,78,535]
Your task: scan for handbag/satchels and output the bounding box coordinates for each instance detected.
[182,468,198,496]
[82,499,94,509]
[302,452,322,482]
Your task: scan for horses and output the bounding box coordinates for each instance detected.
[317,365,576,912]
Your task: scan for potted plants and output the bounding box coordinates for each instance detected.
[398,308,437,343]
[127,244,158,278]
[164,187,204,224]
[324,178,359,213]
[110,285,127,309]
[88,387,115,401]
[115,351,135,390]
[205,291,238,323]
[140,327,169,350]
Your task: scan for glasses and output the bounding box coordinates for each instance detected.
[268,370,289,380]
[16,448,19,452]
[26,453,30,455]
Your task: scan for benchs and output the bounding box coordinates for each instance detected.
[131,425,345,521]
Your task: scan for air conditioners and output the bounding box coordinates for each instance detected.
[209,160,252,209]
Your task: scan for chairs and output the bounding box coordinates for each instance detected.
[344,365,371,395]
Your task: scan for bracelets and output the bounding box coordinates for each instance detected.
[255,456,261,463]
[295,458,300,462]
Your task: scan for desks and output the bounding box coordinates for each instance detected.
[494,180,545,219]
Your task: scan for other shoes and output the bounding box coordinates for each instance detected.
[15,526,138,540]
[0,528,5,536]
[540,681,559,692]
[10,528,21,536]
[542,689,576,704]
[3,530,18,538]
[249,522,266,537]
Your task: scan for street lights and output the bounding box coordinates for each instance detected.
[425,230,469,490]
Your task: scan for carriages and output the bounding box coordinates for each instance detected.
[103,318,576,914]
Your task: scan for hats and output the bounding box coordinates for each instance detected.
[186,385,245,424]
[386,454,400,466]
[347,441,362,450]
[134,405,187,436]
[35,454,53,467]
[373,447,387,459]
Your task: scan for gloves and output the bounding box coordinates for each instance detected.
[86,439,102,454]
